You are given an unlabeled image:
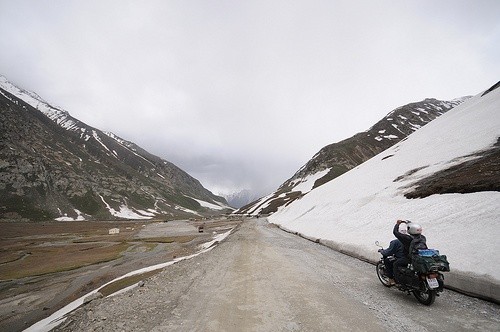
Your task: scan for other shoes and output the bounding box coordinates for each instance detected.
[385,280,396,286]
[394,278,401,284]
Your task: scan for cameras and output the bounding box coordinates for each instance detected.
[401,221,406,223]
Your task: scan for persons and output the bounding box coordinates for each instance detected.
[393,219,426,279]
[379,228,408,279]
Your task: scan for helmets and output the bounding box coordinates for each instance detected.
[407,222,423,234]
[398,223,408,235]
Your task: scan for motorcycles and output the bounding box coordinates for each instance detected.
[375,240,444,307]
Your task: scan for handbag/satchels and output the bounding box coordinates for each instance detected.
[414,254,450,274]
